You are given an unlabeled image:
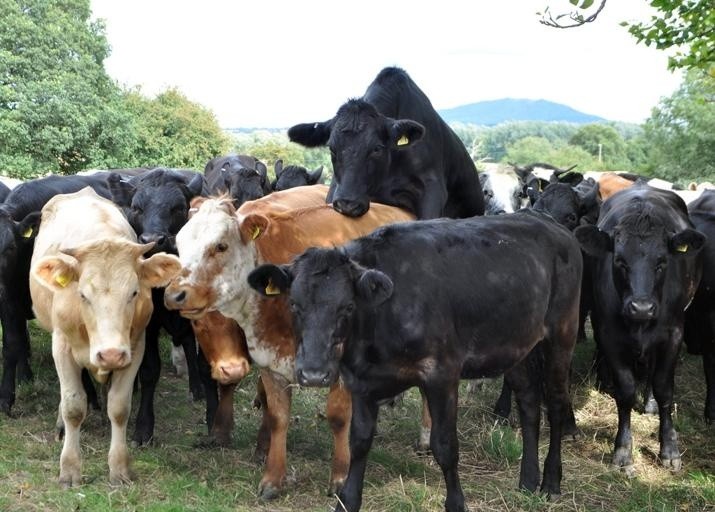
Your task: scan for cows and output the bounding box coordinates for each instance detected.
[292,65,486,221]
[0,152,715,512]
